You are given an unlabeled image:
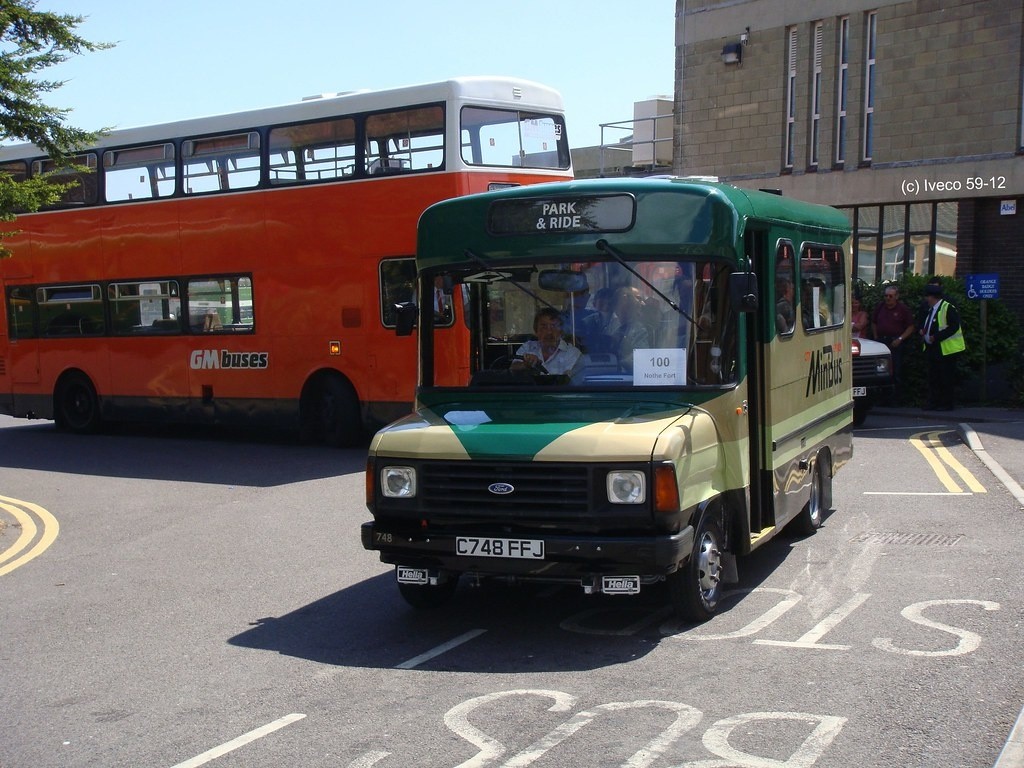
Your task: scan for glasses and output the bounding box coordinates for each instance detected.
[884,295,893,298]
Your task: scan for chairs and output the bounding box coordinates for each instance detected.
[150,313,221,333]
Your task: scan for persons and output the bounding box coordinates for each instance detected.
[433,278,451,321]
[919,277,960,321]
[560,283,663,370]
[919,287,966,410]
[774,279,830,333]
[871,286,914,406]
[850,298,869,338]
[510,309,589,377]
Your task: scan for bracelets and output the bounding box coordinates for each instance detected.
[898,336,904,342]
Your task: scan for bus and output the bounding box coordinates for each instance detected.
[0,82,575,445]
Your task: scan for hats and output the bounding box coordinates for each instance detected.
[924,277,945,292]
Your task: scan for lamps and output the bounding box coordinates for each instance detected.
[720,43,741,65]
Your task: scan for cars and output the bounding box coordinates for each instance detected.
[854,339,895,425]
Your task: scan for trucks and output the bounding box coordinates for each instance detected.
[361,176,855,621]
